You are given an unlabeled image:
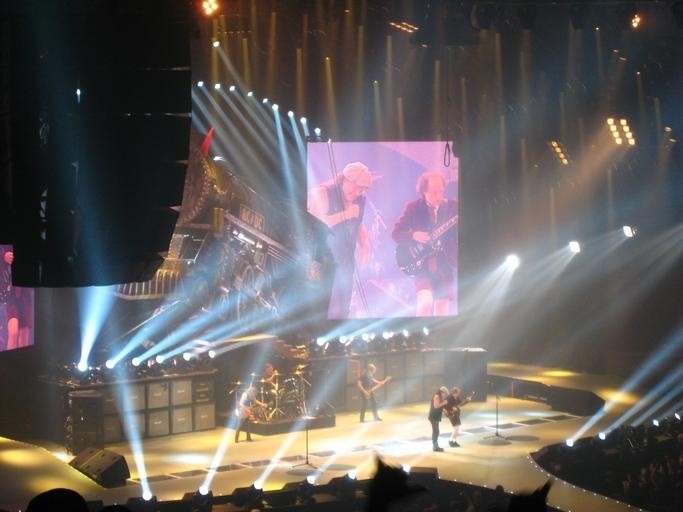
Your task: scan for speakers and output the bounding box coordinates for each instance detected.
[68,390,103,456]
[549,386,605,415]
[410,465,439,480]
[68,446,131,489]
[447,348,488,403]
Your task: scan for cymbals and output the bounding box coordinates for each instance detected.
[232,383,246,384]
[292,372,307,375]
[255,380,270,382]
[292,364,309,369]
[247,372,263,376]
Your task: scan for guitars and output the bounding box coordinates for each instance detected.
[445,395,473,417]
[396,217,457,275]
[239,401,264,418]
[360,376,392,398]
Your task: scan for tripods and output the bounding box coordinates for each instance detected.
[231,386,289,422]
[292,414,319,469]
[483,391,508,441]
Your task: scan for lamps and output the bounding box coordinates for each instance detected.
[553,407,682,457]
[314,325,431,356]
[124,463,361,512]
[71,349,219,378]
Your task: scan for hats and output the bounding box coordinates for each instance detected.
[341,162,373,188]
[439,386,450,396]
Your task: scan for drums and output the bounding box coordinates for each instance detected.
[283,378,298,395]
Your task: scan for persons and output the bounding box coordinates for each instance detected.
[429,386,448,451]
[309,160,371,320]
[444,387,469,449]
[5,252,34,350]
[233,385,268,442]
[261,362,278,395]
[23,489,87,511]
[391,172,458,319]
[356,457,553,512]
[357,363,383,422]
[100,505,132,511]
[621,412,683,511]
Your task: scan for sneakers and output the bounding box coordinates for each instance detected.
[374,417,384,421]
[359,419,366,422]
[449,440,460,448]
[433,447,444,451]
